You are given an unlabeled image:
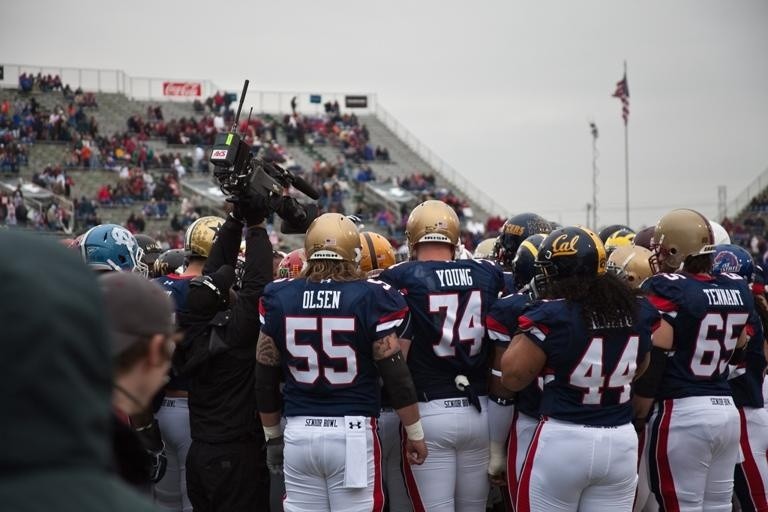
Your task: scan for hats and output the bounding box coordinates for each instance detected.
[186,265,235,319]
[96,272,175,357]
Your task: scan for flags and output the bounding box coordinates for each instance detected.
[614,75,628,121]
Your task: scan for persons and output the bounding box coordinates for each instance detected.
[1,199,768,511]
[1,73,504,251]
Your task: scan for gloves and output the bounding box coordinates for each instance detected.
[266,435,284,475]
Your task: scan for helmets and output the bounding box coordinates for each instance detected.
[462,238,497,260]
[58,224,183,280]
[406,199,460,260]
[235,240,306,287]
[496,212,755,298]
[184,216,226,258]
[304,212,362,264]
[360,232,396,272]
